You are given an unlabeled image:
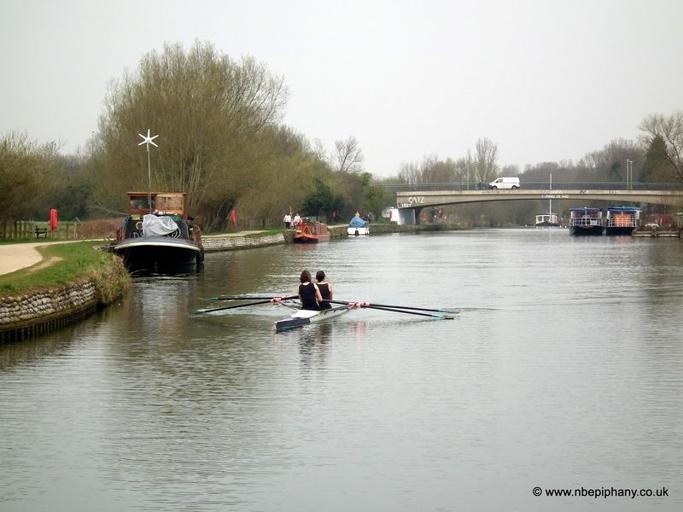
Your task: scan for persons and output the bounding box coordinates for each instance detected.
[297,268,333,312]
[283,212,302,230]
[368,211,376,224]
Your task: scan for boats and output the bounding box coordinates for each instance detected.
[606,206,639,236]
[275,304,352,334]
[292,217,331,243]
[567,206,606,237]
[112,127,205,273]
[347,211,371,235]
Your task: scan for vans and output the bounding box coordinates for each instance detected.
[488,176,520,189]
